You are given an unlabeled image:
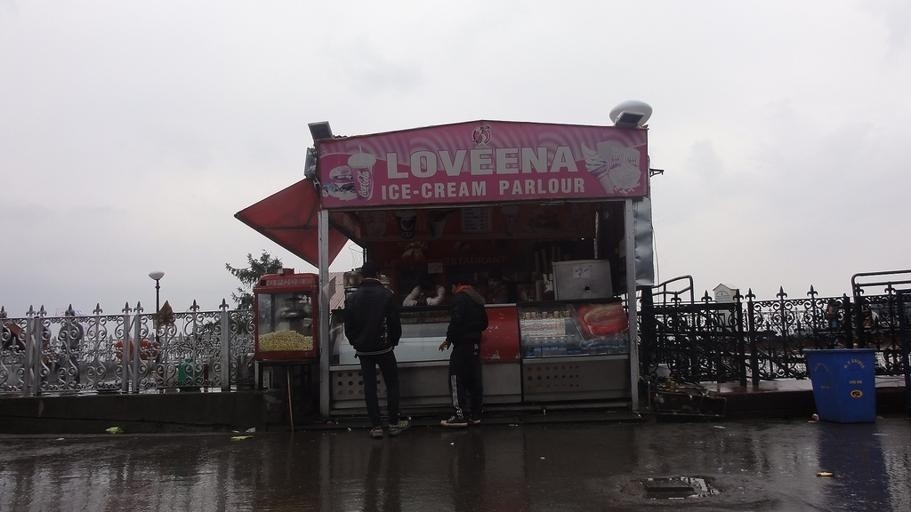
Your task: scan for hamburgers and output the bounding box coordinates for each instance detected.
[329,165,354,198]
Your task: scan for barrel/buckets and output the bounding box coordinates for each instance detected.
[802,346,878,423]
[178,358,202,391]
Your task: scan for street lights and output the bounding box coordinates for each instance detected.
[146,270,168,393]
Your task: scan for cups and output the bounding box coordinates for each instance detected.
[347,153,376,201]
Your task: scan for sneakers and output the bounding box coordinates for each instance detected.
[467,414,480,425]
[440,415,467,427]
[367,427,383,438]
[387,421,408,437]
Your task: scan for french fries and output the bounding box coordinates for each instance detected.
[582,144,603,173]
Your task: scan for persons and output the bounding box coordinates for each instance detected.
[479,269,512,304]
[2,309,162,393]
[402,271,447,307]
[438,275,490,427]
[344,262,414,438]
[825,297,883,347]
